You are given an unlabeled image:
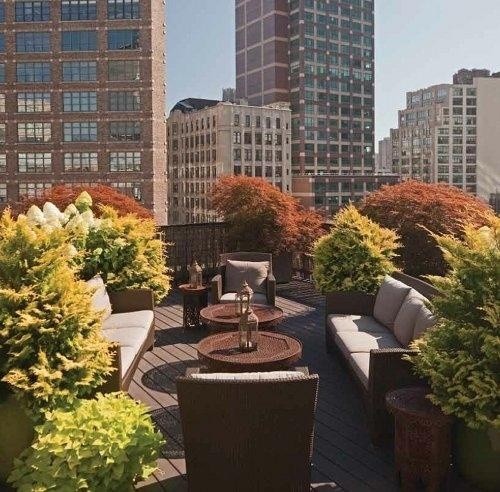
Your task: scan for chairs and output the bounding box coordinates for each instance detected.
[175,364,320,490]
[209,251,275,307]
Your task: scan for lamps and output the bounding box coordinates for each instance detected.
[237,305,259,353]
[234,280,254,316]
[186,260,205,287]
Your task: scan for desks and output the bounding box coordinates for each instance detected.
[196,331,305,371]
[384,385,456,491]
[180,283,208,331]
[199,301,284,334]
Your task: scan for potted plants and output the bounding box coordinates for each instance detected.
[402,203,500,492]
[1,203,125,478]
[207,174,326,284]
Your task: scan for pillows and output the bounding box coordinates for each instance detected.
[414,302,439,342]
[224,258,268,293]
[373,274,411,325]
[391,290,422,345]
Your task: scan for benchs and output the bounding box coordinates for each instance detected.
[83,271,157,397]
[323,269,450,447]
